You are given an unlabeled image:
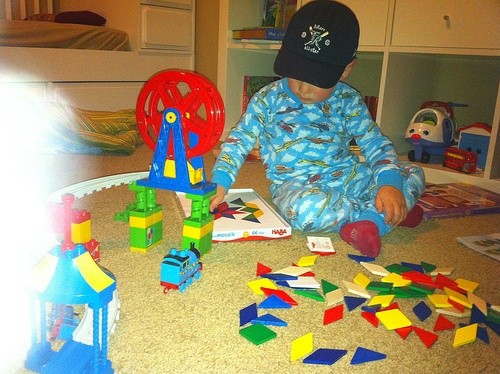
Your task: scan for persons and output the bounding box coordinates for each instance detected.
[210,0,426,256]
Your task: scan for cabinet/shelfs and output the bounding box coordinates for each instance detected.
[0,0,500,194]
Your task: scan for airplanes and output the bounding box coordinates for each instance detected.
[404,99,469,163]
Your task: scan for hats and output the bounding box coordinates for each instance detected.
[273,0,360,88]
